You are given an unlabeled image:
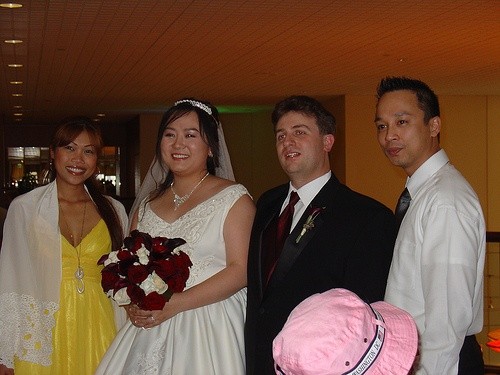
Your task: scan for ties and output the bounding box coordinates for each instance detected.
[395,187,412,240]
[267,191,300,285]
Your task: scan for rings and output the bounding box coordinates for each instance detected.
[151,317,154,322]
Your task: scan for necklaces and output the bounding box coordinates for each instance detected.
[168,172,211,212]
[55,192,88,295]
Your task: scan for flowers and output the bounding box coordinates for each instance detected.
[97,230,193,311]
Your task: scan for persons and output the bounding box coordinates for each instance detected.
[93,95,256,375]
[93,179,124,199]
[375,73,489,375]
[242,95,401,375]
[1,117,131,375]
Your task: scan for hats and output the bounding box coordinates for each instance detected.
[273,288,418,375]
